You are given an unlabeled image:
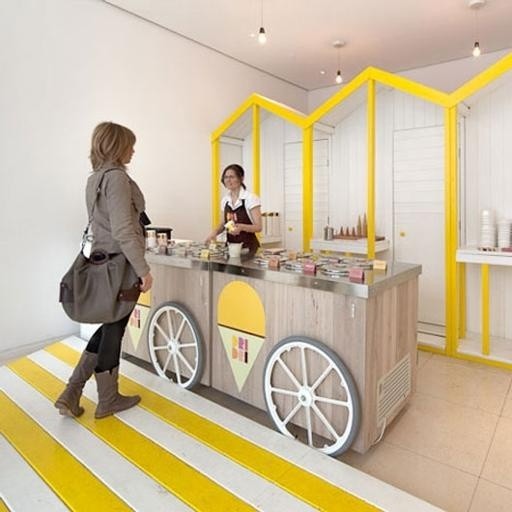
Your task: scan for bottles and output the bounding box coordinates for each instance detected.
[324,228,333,240]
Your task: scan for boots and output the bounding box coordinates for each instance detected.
[54,349,98,417]
[95,363,141,418]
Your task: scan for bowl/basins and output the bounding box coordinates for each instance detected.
[144,230,167,251]
[479,207,512,248]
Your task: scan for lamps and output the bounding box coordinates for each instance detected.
[468,0,487,58]
[258,1,267,46]
[333,41,345,84]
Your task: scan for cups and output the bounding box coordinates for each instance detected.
[225,221,237,234]
[228,243,241,258]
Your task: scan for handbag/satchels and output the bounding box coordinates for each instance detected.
[59,252,140,324]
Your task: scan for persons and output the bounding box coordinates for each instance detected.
[54,122,154,419]
[205,164,263,253]
